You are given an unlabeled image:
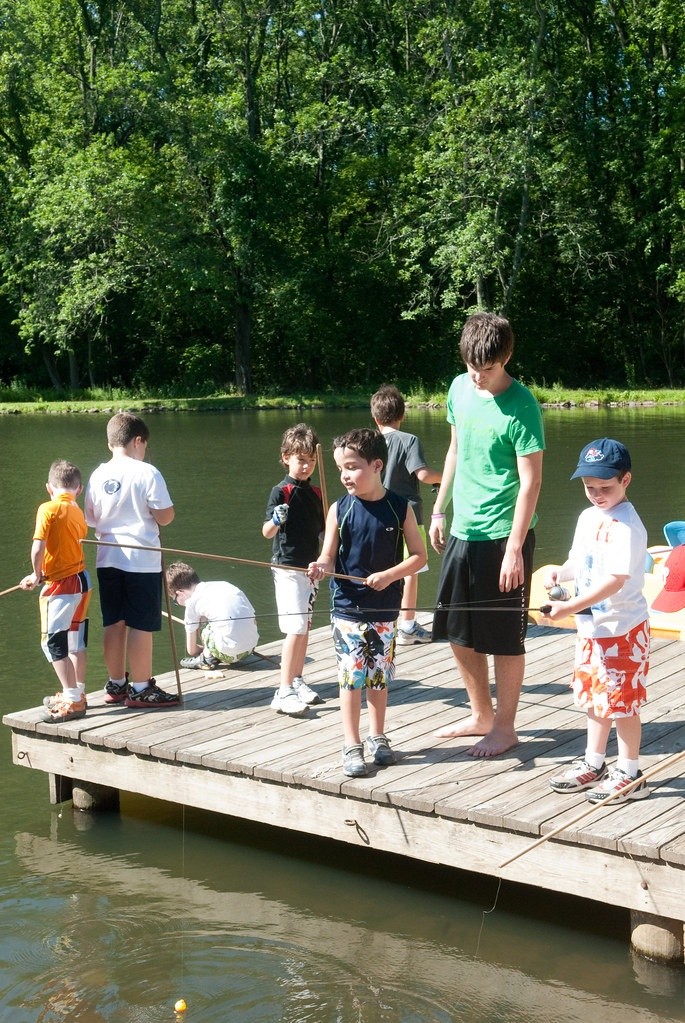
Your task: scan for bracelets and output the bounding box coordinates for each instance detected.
[431,513,446,518]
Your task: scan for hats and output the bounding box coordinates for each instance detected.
[570,436,631,480]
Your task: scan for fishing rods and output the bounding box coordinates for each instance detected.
[161,610,279,665]
[316,442,327,521]
[430,482,441,505]
[0,575,48,596]
[185,604,592,625]
[76,536,370,584]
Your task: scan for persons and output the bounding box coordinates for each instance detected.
[165,561,260,670]
[306,428,428,776]
[429,310,542,757]
[263,423,326,714]
[370,386,443,643]
[542,437,650,805]
[20,459,91,723]
[84,413,179,706]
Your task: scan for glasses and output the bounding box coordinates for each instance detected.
[173,590,184,606]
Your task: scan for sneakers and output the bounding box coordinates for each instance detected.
[104,672,133,702]
[585,768,649,804]
[397,620,433,644]
[550,761,609,793]
[366,733,396,765]
[180,652,215,669]
[271,687,310,713]
[342,742,368,776]
[292,676,320,703]
[124,677,179,706]
[43,691,87,709]
[39,693,87,723]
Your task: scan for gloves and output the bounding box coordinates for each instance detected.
[272,503,289,526]
